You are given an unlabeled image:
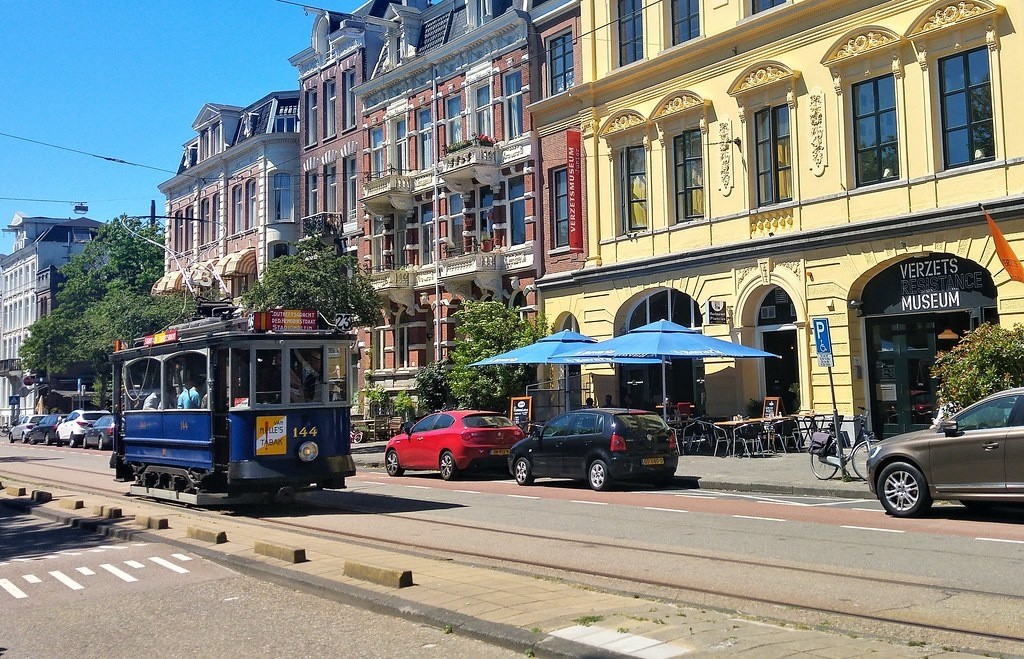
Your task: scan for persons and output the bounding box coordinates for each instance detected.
[584,397,594,408]
[603,395,617,408]
[130,352,333,410]
[930,398,955,430]
[624,393,638,408]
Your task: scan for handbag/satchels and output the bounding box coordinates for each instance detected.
[808,431,832,455]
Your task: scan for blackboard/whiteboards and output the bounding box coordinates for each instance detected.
[760,397,786,440]
[510,396,532,439]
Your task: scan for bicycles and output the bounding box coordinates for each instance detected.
[0,422,15,437]
[809,406,881,482]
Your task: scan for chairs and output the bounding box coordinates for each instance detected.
[665,401,849,460]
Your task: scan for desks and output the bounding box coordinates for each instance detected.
[790,413,833,448]
[656,404,695,420]
[713,418,770,459]
[748,415,795,455]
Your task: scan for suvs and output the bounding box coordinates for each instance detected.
[55,408,111,448]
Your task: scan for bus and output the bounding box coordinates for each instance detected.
[108,215,359,507]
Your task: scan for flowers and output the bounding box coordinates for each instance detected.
[479,134,496,144]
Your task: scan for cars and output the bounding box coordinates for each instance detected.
[9,415,50,444]
[82,415,125,451]
[508,407,680,491]
[863,387,1024,518]
[27,413,70,446]
[385,410,525,481]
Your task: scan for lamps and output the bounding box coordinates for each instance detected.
[73,202,88,214]
[848,299,863,309]
[340,17,366,39]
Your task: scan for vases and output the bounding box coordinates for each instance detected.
[479,139,493,147]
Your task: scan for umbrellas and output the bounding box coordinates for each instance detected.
[467,328,671,413]
[548,318,782,422]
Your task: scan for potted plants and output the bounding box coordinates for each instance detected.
[480,231,491,252]
[447,138,472,153]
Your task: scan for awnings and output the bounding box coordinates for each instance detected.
[151,247,256,294]
